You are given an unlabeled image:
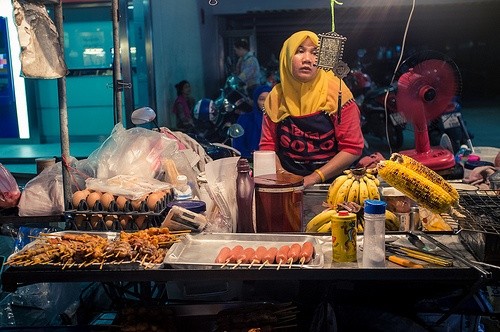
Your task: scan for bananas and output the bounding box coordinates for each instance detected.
[304,168,400,233]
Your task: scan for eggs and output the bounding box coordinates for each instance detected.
[73,187,171,229]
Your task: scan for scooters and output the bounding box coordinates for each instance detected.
[429,97,475,142]
[131,73,254,161]
[346,70,408,150]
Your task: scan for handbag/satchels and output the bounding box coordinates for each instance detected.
[0,163,21,208]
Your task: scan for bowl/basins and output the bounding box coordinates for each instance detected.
[381,187,414,213]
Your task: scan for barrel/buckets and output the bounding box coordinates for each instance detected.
[468,146,500,166]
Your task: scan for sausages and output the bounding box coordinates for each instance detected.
[214,240,314,265]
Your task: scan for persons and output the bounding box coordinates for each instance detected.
[174,80,206,133]
[230,83,272,161]
[259,31,365,190]
[232,40,260,100]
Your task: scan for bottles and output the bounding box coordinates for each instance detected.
[236,158,256,233]
[455,145,468,162]
[409,207,420,231]
[464,156,482,179]
[173,175,192,200]
[331,211,357,267]
[362,199,387,267]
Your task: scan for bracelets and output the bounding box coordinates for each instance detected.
[315,169,326,183]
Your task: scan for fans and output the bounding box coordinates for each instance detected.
[388,50,463,171]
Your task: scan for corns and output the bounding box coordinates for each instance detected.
[376,154,461,214]
